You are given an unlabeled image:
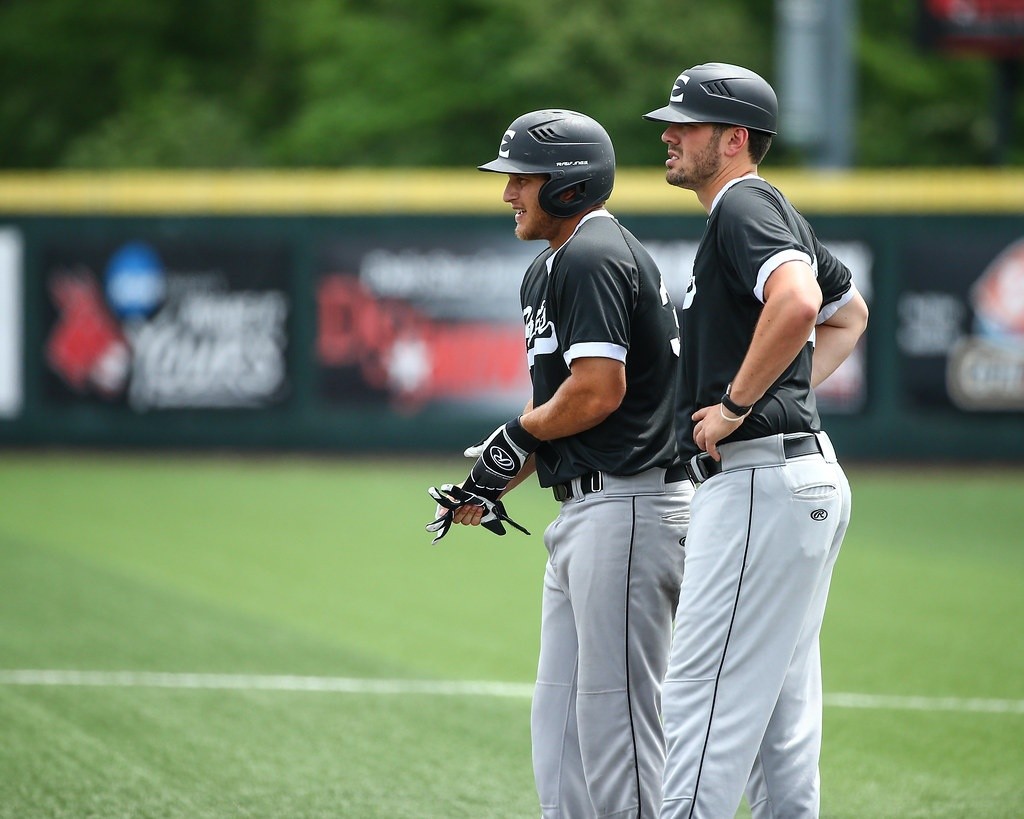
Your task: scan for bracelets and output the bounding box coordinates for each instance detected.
[721,403,752,421]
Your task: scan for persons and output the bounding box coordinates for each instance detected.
[644,63,870,819]
[454,109,692,819]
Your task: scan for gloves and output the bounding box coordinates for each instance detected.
[458,414,543,502]
[424,485,531,545]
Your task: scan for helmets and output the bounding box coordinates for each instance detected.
[477,108,615,217]
[642,62,778,136]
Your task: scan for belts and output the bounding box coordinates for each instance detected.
[684,435,821,483]
[553,463,688,501]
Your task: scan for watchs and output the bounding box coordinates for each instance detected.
[720,382,751,417]
